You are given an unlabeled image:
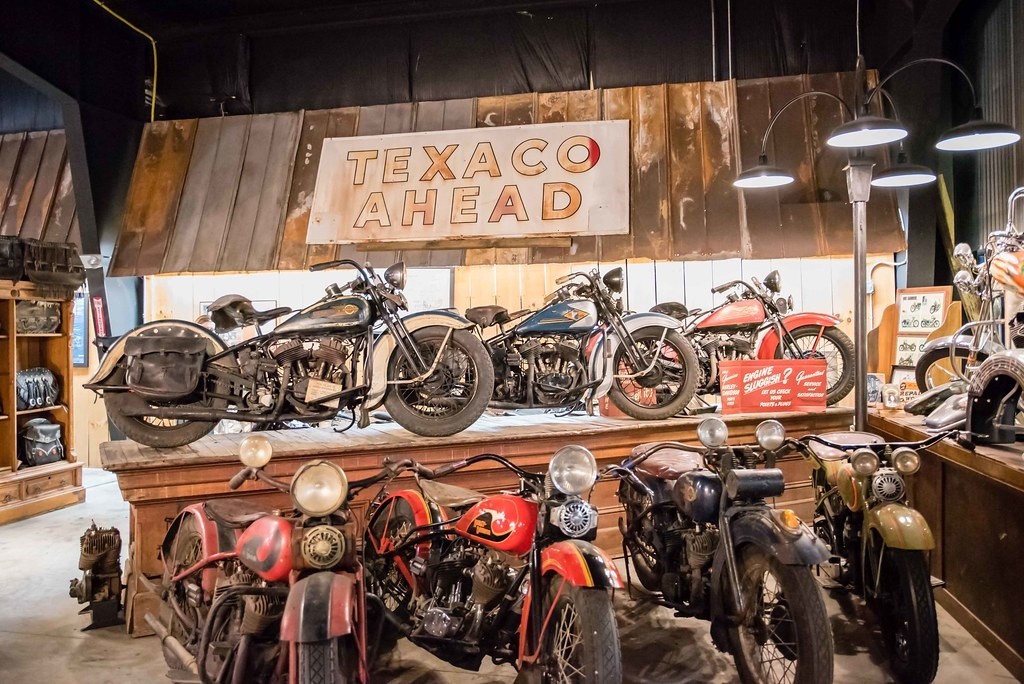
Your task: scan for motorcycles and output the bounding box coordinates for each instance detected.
[403,267,700,423]
[613,432,845,682]
[586,271,857,413]
[914,185,1024,442]
[368,444,655,683]
[163,438,432,684]
[92,262,498,452]
[758,422,976,682]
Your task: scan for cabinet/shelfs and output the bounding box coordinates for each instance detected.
[0,278,85,527]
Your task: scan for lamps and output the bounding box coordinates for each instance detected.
[734,58,1020,434]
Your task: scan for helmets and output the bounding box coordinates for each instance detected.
[206,295,255,334]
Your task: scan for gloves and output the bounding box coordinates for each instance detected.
[244,305,291,326]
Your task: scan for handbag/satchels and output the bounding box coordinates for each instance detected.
[17,417,65,466]
[467,305,510,328]
[17,299,60,334]
[124,334,207,401]
[16,367,59,411]
[649,302,688,320]
[0,233,86,291]
